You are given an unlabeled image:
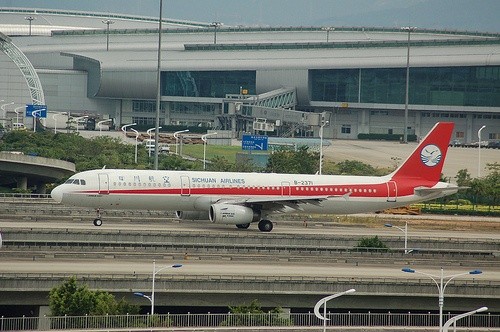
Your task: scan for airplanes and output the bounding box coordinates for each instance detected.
[50,120,472,231]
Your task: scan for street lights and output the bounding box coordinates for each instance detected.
[313,288,356,332]
[52,111,67,135]
[101,19,117,54]
[14,105,29,132]
[32,108,46,133]
[24,15,37,37]
[201,131,218,171]
[1,102,15,129]
[74,115,89,137]
[319,120,329,175]
[321,26,337,42]
[147,126,162,158]
[121,122,137,134]
[478,125,486,179]
[173,129,189,153]
[208,20,223,47]
[401,266,488,332]
[398,25,418,144]
[97,119,113,137]
[133,261,183,316]
[130,128,139,165]
[384,222,420,255]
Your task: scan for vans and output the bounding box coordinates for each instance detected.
[13,122,24,128]
[142,138,156,153]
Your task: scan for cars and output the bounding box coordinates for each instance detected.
[448,140,500,149]
